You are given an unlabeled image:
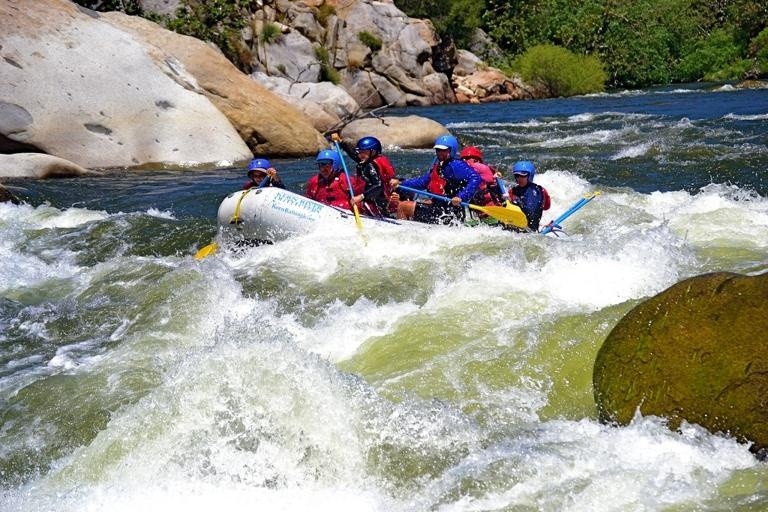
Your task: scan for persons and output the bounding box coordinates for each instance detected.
[460,146,501,218]
[389,136,481,225]
[503,161,550,233]
[332,132,396,216]
[304,150,366,213]
[243,159,285,189]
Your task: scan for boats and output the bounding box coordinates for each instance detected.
[218,187,571,255]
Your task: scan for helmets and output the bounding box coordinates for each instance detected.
[432,135,458,158]
[246,159,273,178]
[313,149,340,174]
[512,160,535,183]
[353,136,382,154]
[459,146,483,163]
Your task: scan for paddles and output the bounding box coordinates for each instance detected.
[397,182,528,229]
[332,140,361,227]
[494,176,522,211]
[188,175,272,262]
